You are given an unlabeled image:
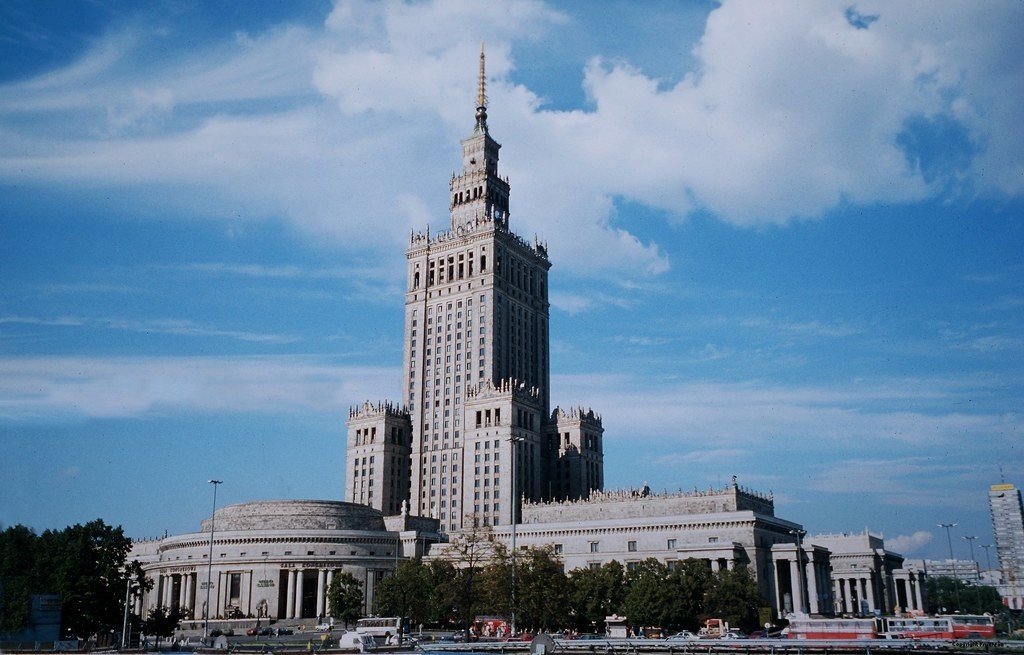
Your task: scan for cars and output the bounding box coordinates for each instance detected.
[451,630,479,643]
[676,629,702,641]
[390,634,418,646]
[501,633,600,647]
[315,622,335,630]
[246,626,272,636]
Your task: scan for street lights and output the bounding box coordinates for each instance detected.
[505,435,526,639]
[979,543,998,616]
[788,528,807,613]
[961,534,982,615]
[203,478,224,637]
[934,521,962,612]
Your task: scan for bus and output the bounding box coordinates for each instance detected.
[355,616,410,636]
[885,613,997,638]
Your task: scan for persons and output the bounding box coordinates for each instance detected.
[316,612,323,626]
[80,609,706,651]
